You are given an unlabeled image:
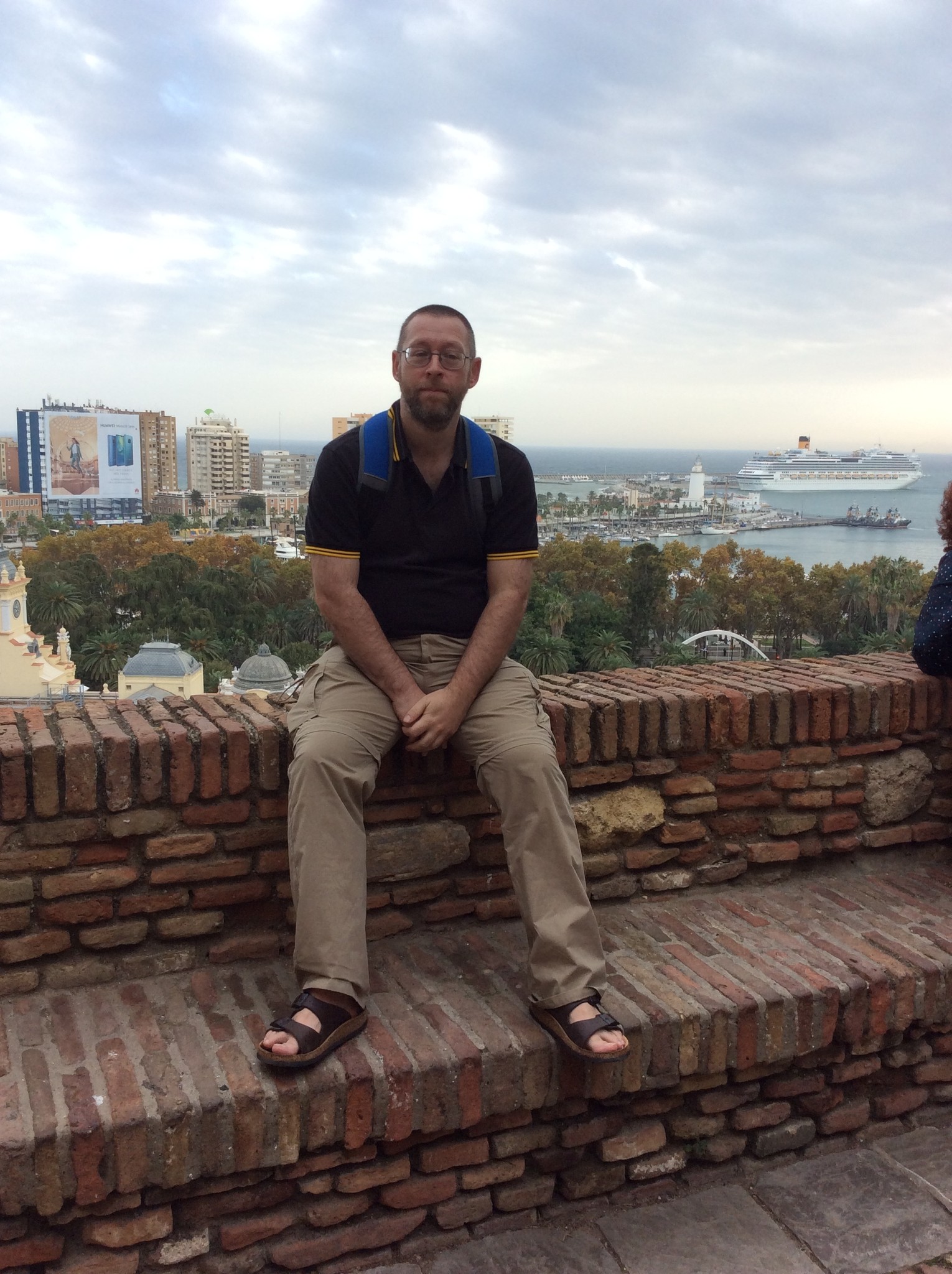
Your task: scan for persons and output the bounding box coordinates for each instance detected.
[255,304,632,1068]
[912,480,952,678]
[66,438,85,478]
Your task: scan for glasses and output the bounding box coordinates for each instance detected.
[398,347,473,370]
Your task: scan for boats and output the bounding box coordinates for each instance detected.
[836,474,844,480]
[876,474,884,479]
[261,539,306,562]
[710,476,729,484]
[798,473,807,479]
[828,474,834,479]
[892,474,898,479]
[860,474,868,479]
[790,474,798,479]
[845,474,852,479]
[852,474,859,479]
[819,474,827,479]
[808,474,815,479]
[868,474,875,479]
[829,502,912,528]
[884,474,891,479]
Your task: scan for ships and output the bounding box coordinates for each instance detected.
[735,436,923,491]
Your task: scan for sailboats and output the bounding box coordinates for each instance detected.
[614,516,650,542]
[658,497,679,537]
[701,479,739,535]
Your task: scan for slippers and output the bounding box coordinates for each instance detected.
[529,998,631,1062]
[257,994,367,1068]
